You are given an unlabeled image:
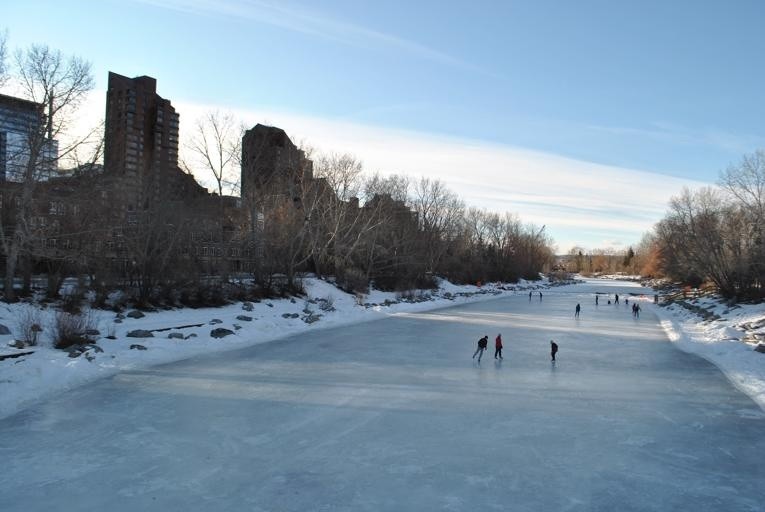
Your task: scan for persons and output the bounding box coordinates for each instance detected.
[575,303,580,317]
[549,340,558,362]
[540,292,542,296]
[595,294,642,318]
[529,292,532,297]
[472,335,489,362]
[494,333,503,359]
